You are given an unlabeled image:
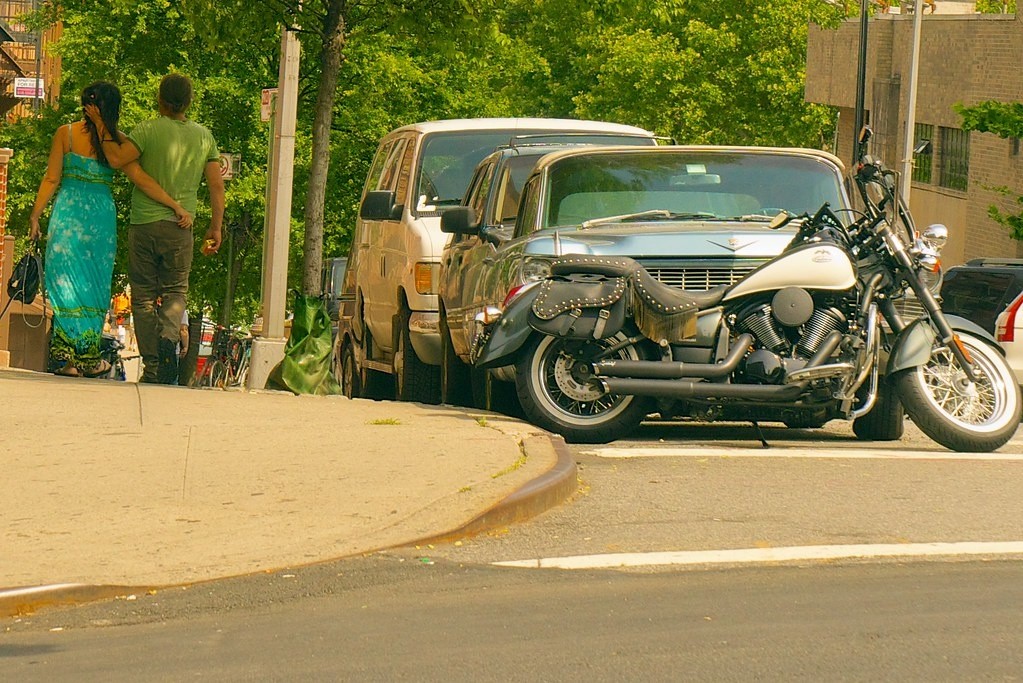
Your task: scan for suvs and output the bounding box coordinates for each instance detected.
[437,140,595,406]
[940,258,1023,392]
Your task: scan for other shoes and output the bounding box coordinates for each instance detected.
[140,376,157,383]
[158,338,177,383]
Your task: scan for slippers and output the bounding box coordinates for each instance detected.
[54,358,111,378]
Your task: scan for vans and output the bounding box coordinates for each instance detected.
[336,118,658,404]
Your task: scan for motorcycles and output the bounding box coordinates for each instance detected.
[472,126,1023,454]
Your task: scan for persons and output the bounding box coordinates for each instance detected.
[84,73,224,384]
[172,310,189,385]
[29,81,192,377]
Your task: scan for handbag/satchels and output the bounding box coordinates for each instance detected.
[6,234,39,304]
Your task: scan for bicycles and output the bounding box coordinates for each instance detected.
[196,320,248,389]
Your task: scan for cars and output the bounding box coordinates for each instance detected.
[325,255,349,327]
[444,145,909,441]
